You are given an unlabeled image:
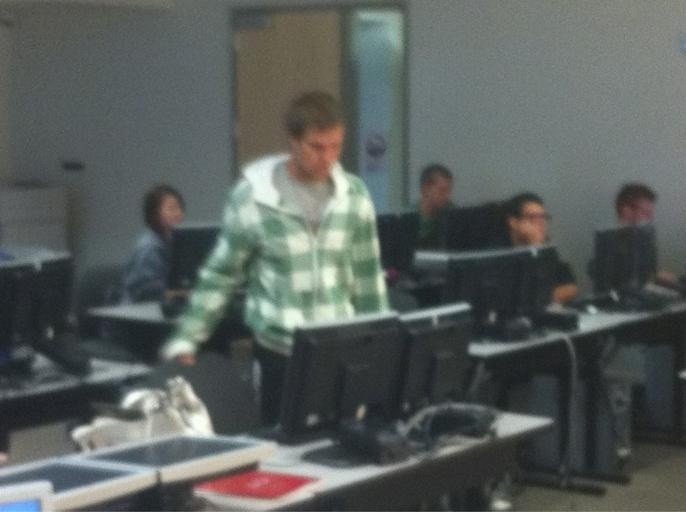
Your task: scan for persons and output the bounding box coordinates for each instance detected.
[389,156,464,316]
[162,87,389,429]
[120,183,186,306]
[589,181,686,449]
[449,191,633,487]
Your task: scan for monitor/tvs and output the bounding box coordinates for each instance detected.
[164,224,225,291]
[278,312,412,448]
[442,246,559,325]
[0,478,56,512]
[591,222,658,295]
[446,203,508,255]
[398,302,475,420]
[376,211,422,278]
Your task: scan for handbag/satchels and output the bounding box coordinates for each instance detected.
[67,376,214,453]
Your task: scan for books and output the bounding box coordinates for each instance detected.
[194,467,328,506]
